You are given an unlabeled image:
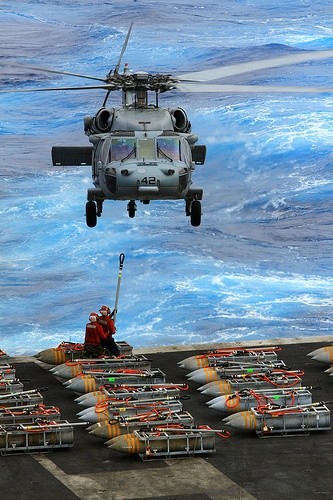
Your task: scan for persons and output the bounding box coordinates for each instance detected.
[85,312,106,357]
[95,305,119,356]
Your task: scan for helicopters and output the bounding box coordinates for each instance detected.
[1,22,333,229]
